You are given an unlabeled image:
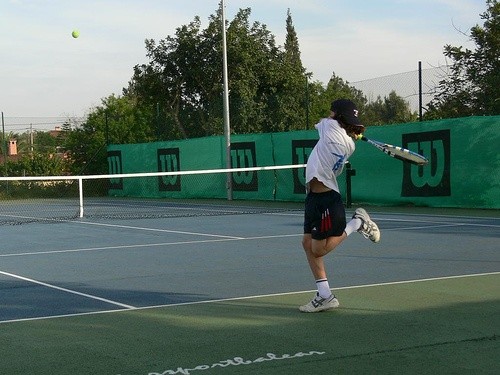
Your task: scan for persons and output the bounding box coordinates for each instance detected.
[298,99,380,313]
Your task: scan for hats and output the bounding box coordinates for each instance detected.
[331,98,364,127]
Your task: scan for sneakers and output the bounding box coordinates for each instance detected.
[352,208,380,243]
[299,292,339,313]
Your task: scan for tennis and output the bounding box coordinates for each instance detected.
[72,30,79,38]
